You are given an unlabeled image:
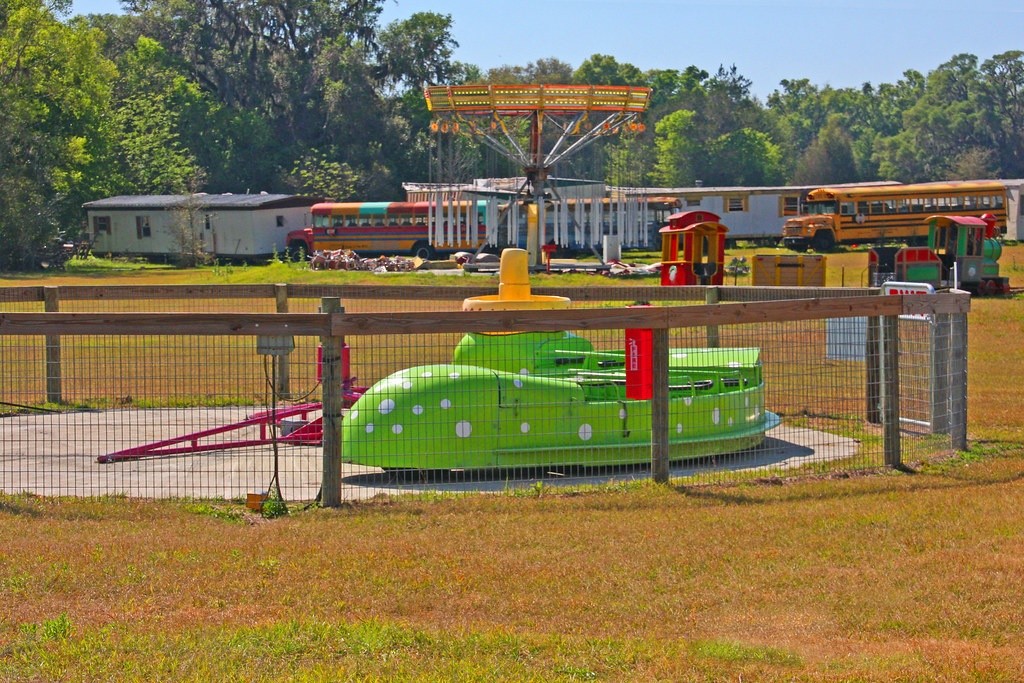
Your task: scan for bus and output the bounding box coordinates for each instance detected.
[782,181,1009,252]
[500,195,683,252]
[284,200,493,263]
